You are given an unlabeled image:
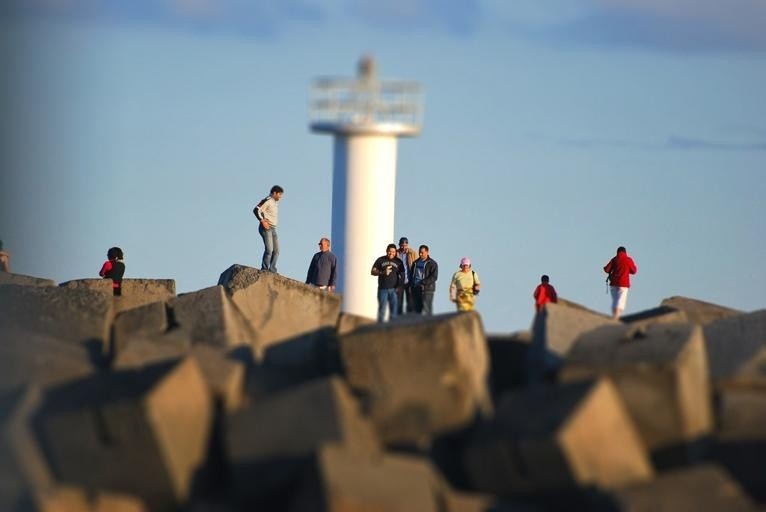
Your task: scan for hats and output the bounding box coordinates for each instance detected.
[460,257,471,266]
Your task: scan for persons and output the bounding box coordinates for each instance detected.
[534,274,559,316]
[369,243,404,322]
[410,243,437,317]
[395,237,416,315]
[450,257,482,314]
[303,237,337,293]
[100,246,125,297]
[603,245,638,321]
[0,239,9,273]
[253,185,283,275]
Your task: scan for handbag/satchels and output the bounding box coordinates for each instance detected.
[472,271,479,295]
